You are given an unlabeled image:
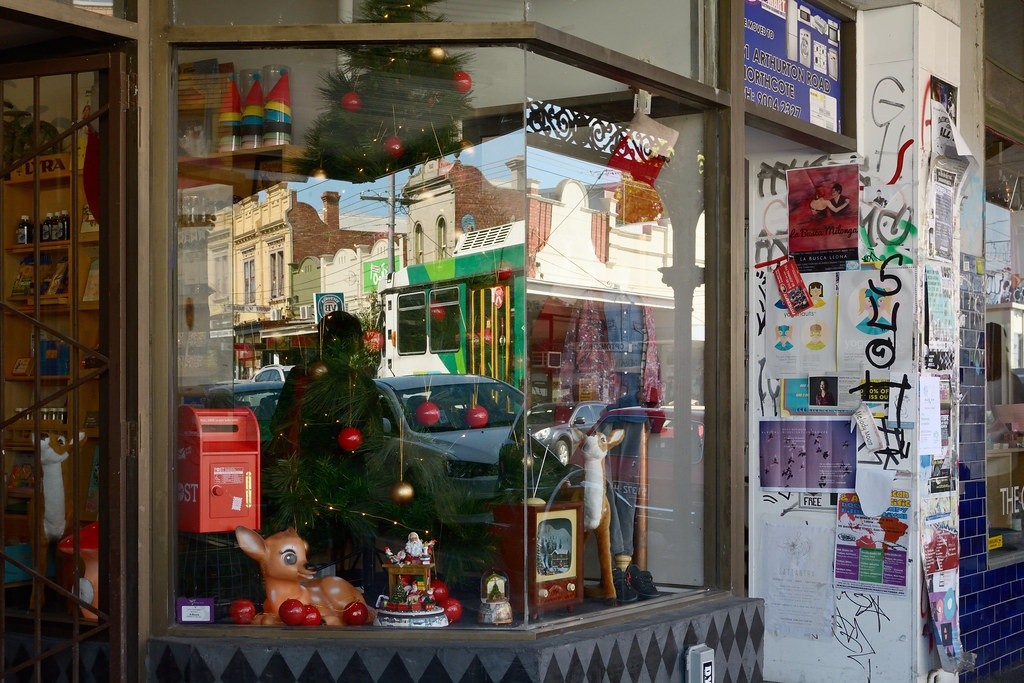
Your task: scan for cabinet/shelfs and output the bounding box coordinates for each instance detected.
[528,313,604,403]
[0,149,103,600]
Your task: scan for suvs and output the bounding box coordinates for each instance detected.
[242,364,296,405]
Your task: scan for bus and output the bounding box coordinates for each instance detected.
[374,243,526,395]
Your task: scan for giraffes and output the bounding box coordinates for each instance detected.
[27,431,87,611]
[565,428,625,600]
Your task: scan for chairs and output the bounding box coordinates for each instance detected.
[254,394,279,418]
[407,396,440,431]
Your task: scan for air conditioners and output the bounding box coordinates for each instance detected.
[531,351,562,368]
[270,309,282,321]
[299,306,313,319]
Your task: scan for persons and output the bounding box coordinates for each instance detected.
[268,312,383,583]
[827,183,850,216]
[816,381,834,406]
[873,189,887,206]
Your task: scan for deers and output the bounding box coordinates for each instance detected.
[235,526,377,626]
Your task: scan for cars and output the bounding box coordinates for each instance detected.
[951,370,1024,453]
[523,400,611,466]
[371,374,526,528]
[208,383,290,510]
[655,399,704,439]
[567,407,706,567]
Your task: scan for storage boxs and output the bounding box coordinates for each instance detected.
[532,377,598,403]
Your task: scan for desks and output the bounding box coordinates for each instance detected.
[987,449,1024,528]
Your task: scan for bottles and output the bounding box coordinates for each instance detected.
[10,406,67,424]
[17,214,31,243]
[40,209,71,241]
